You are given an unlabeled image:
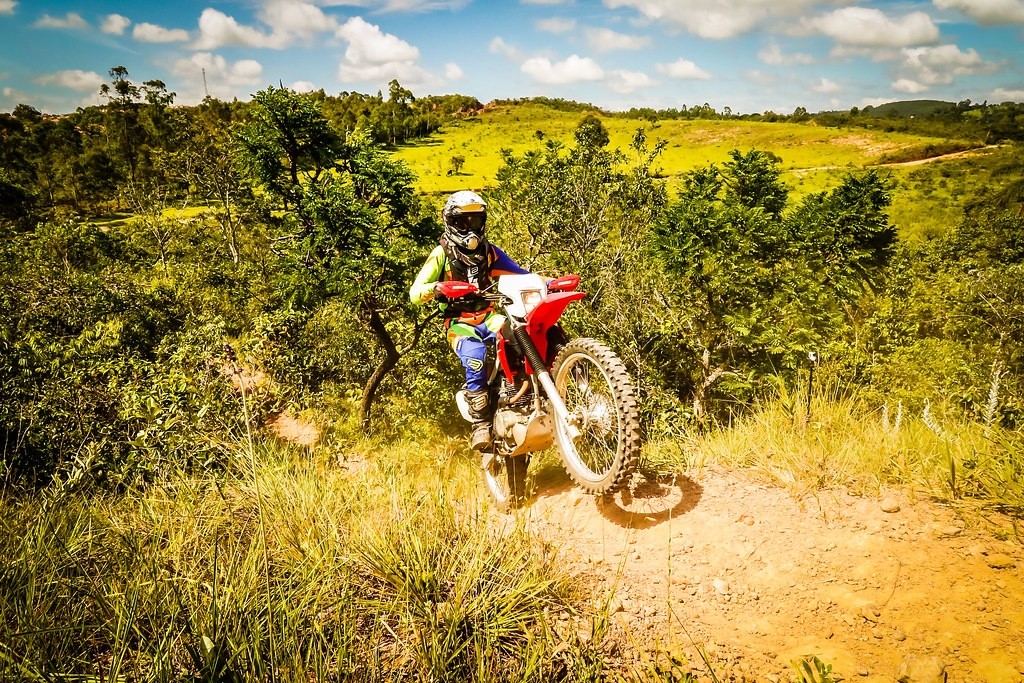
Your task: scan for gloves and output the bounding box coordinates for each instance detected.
[546,275,579,291]
[437,281,478,298]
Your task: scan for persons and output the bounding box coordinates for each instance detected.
[408,190,567,451]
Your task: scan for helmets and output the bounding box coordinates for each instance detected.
[443,190,487,250]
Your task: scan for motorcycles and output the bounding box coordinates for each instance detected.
[437,274,641,515]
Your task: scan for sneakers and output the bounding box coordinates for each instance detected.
[471,422,492,450]
